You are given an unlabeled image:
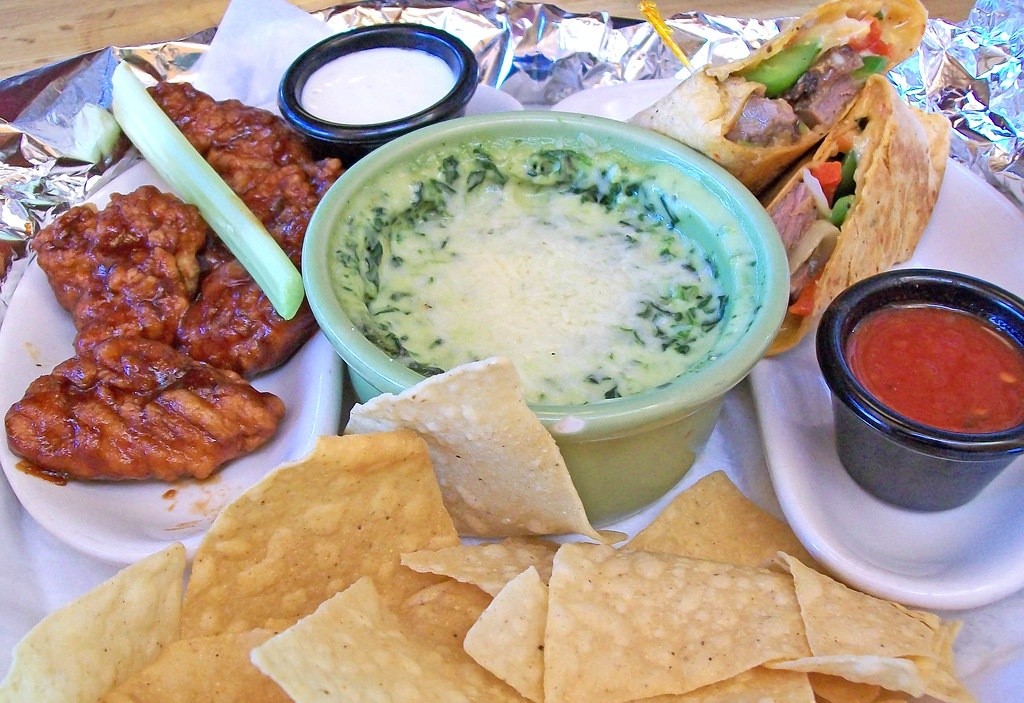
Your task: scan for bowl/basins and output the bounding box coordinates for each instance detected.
[813,264,1024,513]
[275,21,480,166]
[302,108,791,534]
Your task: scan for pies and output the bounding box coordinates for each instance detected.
[629,0,950,352]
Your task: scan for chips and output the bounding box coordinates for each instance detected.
[2,357,984,703]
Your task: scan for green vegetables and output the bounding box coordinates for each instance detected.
[338,149,730,400]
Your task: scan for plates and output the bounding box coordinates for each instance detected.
[0,159,344,571]
[547,78,691,124]
[744,156,1024,613]
[463,82,526,115]
